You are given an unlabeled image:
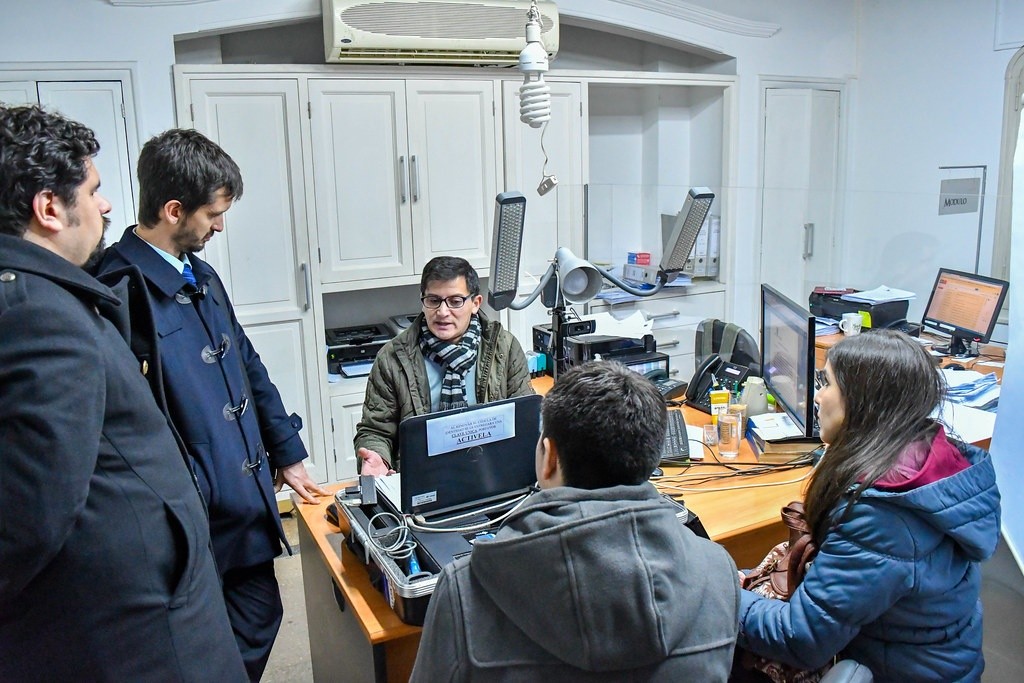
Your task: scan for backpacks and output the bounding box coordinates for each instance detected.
[735,501,831,683]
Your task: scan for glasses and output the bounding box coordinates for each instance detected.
[420,293,477,309]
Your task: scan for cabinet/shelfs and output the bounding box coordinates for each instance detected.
[0,60,149,265]
[166,63,739,514]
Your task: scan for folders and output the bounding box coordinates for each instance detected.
[624,264,694,287]
[684,214,721,277]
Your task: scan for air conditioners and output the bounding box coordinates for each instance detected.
[320,0,559,67]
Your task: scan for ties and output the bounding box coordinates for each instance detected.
[181,264,196,286]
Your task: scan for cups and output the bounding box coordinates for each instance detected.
[704,425,718,446]
[719,416,737,444]
[718,408,741,458]
[839,313,862,337]
[729,404,746,440]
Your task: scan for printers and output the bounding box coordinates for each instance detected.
[324,323,398,379]
[808,290,909,334]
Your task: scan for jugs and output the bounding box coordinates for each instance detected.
[740,376,768,426]
[771,376,794,404]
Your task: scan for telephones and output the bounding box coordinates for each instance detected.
[685,352,752,415]
[881,318,925,338]
[640,368,687,401]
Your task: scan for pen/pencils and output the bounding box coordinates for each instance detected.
[711,373,720,390]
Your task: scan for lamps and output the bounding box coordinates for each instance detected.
[486,190,555,313]
[554,247,605,307]
[587,187,716,296]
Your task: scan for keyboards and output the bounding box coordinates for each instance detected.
[658,409,690,464]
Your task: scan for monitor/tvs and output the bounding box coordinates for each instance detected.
[921,267,1010,357]
[758,283,826,446]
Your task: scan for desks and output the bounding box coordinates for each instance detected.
[290,377,998,683]
[813,325,1008,414]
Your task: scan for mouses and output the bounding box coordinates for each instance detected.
[649,467,664,480]
[944,363,965,371]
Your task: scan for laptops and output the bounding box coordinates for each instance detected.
[373,394,545,521]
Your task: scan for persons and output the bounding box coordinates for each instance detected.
[354,255,538,479]
[409,361,741,683]
[0,102,250,683]
[739,325,1003,683]
[96,131,334,683]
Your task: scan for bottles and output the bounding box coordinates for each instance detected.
[731,394,738,404]
